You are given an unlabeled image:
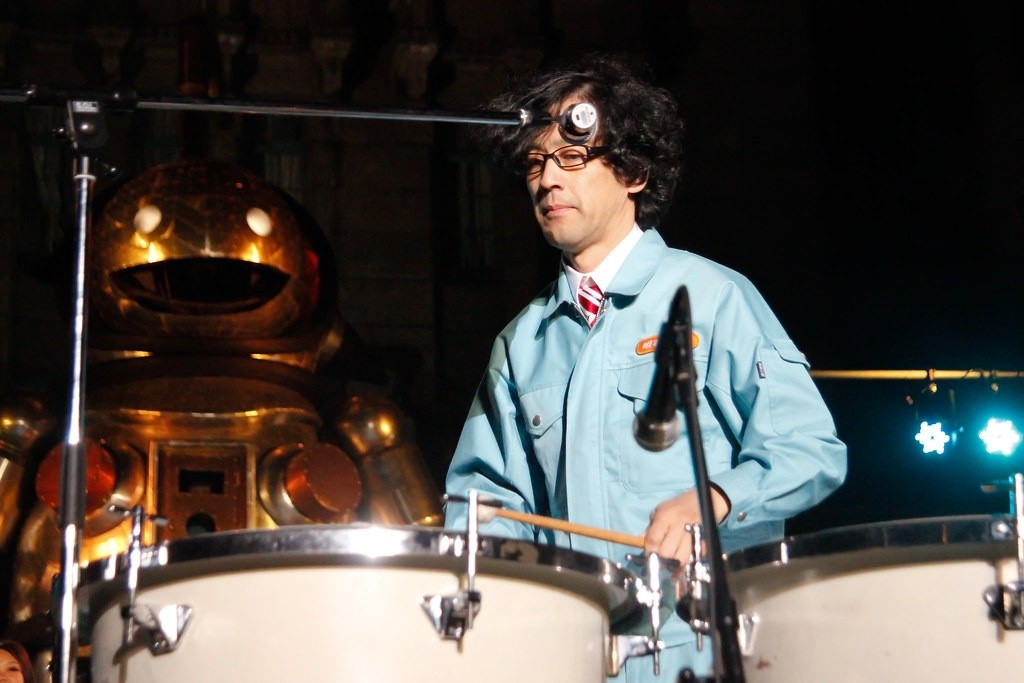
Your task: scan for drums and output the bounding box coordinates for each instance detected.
[48,522,652,683]
[709,515,1024,683]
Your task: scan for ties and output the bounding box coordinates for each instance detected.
[578,284,604,328]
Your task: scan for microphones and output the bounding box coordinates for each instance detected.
[558,103,597,145]
[633,285,690,452]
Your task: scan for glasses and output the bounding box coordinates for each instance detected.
[510,145,609,175]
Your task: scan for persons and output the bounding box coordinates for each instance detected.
[446,52,848,683]
